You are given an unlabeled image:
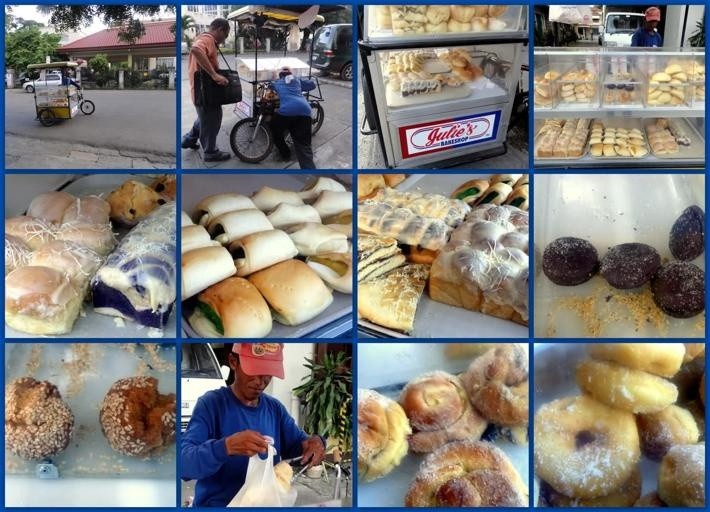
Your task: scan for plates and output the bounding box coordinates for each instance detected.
[424,58,453,74]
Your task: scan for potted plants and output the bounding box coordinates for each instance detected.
[292,350,353,452]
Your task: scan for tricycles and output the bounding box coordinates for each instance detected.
[28,61,96,127]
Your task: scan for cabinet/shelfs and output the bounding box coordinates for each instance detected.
[34,84,78,119]
[532,48,707,169]
[356,4,530,169]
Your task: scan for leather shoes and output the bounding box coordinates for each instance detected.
[182,139,200,149]
[205,151,230,161]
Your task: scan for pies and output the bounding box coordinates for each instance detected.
[181,176,529,338]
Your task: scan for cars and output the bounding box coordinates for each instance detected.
[15,70,40,83]
[22,74,81,94]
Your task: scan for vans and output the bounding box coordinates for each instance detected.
[306,24,354,83]
[179,342,231,434]
[600,12,648,49]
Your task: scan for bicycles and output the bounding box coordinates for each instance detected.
[226,80,323,163]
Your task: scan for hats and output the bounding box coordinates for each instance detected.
[279,66,291,73]
[232,343,285,379]
[645,7,661,22]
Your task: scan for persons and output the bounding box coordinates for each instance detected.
[267,66,316,169]
[181,17,232,162]
[632,7,662,47]
[63,68,81,90]
[179,343,326,508]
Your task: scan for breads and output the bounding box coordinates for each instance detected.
[534,59,705,158]
[358,343,707,507]
[5,174,177,335]
[5,377,73,460]
[377,5,509,97]
[99,376,176,458]
[542,205,704,319]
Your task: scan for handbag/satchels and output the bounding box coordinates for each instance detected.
[194,69,242,107]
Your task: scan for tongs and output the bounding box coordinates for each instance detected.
[282,453,312,484]
